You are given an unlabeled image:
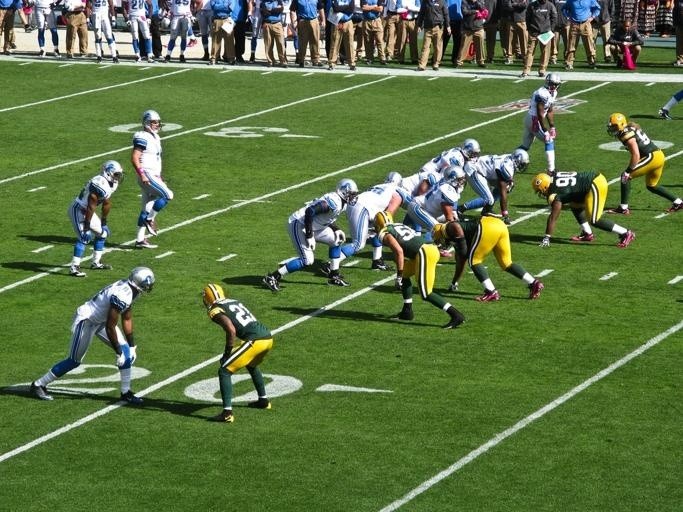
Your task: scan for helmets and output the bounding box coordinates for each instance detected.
[161,17,171,28]
[431,223,453,251]
[128,268,154,292]
[101,160,124,184]
[143,111,163,134]
[336,178,359,205]
[532,172,552,199]
[606,112,627,136]
[388,171,404,186]
[444,139,480,186]
[203,284,225,306]
[374,211,393,232]
[545,73,560,91]
[512,148,529,173]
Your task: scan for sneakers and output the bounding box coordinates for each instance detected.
[480,208,495,216]
[136,53,185,63]
[119,390,142,404]
[319,263,350,287]
[31,381,54,402]
[439,251,452,258]
[209,410,234,424]
[443,314,465,329]
[548,169,556,175]
[391,309,414,320]
[518,56,613,76]
[666,200,682,212]
[97,51,120,64]
[458,205,466,218]
[270,55,389,70]
[249,398,271,410]
[416,59,515,70]
[134,218,158,249]
[528,279,543,299]
[263,274,281,290]
[370,259,390,270]
[41,49,87,60]
[188,37,197,47]
[202,54,256,65]
[673,56,683,65]
[394,278,404,292]
[3,41,16,55]
[658,107,672,119]
[474,289,500,301]
[69,259,111,277]
[569,228,634,247]
[605,204,630,214]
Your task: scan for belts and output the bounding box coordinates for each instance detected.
[338,19,351,23]
[212,16,227,20]
[265,20,278,24]
[366,16,379,21]
[0,6,14,10]
[298,17,316,21]
[400,18,416,22]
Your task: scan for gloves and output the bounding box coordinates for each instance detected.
[190,17,198,22]
[100,227,110,238]
[448,283,461,290]
[86,22,93,26]
[507,181,515,192]
[621,171,632,184]
[83,229,92,242]
[541,234,550,248]
[129,345,138,364]
[307,236,317,249]
[503,213,510,224]
[115,351,125,367]
[112,20,131,28]
[335,229,345,242]
[25,25,33,30]
[544,128,556,142]
[220,352,231,365]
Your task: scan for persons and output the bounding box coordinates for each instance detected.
[519,75,563,174]
[373,210,465,328]
[531,170,636,248]
[30,265,155,404]
[259,136,543,303]
[658,90,683,121]
[132,110,173,248]
[606,113,683,214]
[68,160,124,277]
[203,283,273,423]
[0,0,682,80]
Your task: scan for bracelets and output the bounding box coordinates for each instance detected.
[138,168,143,176]
[625,167,633,174]
